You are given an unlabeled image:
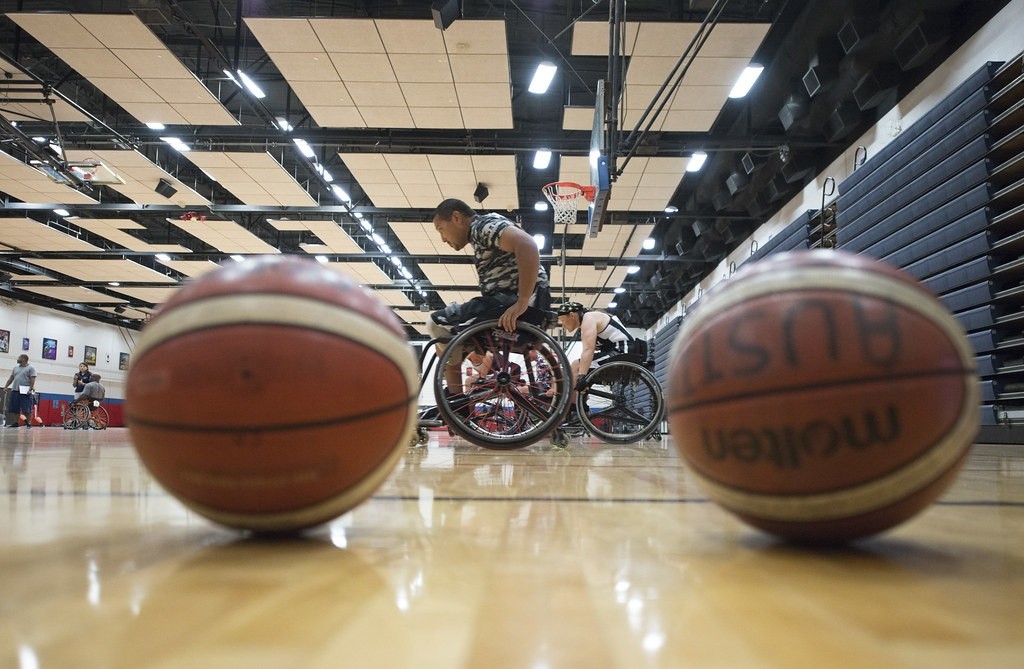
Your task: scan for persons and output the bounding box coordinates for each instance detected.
[464,350,520,418]
[66,374,105,424]
[543,302,636,413]
[417,198,551,428]
[73,362,92,399]
[3,354,36,429]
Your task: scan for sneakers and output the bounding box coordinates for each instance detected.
[417,388,469,419]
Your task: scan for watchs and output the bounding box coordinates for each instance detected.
[29,388,33,390]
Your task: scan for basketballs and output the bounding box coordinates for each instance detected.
[665,248,979,546]
[123,252,420,534]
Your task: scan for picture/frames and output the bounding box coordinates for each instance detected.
[119,352,130,371]
[84,345,97,366]
[42,338,57,360]
[0,329,10,354]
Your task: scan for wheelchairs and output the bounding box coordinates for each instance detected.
[459,378,529,440]
[61,398,110,430]
[548,337,666,444]
[405,277,574,449]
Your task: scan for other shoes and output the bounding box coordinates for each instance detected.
[39,423,45,429]
[26,424,31,429]
[9,422,20,428]
